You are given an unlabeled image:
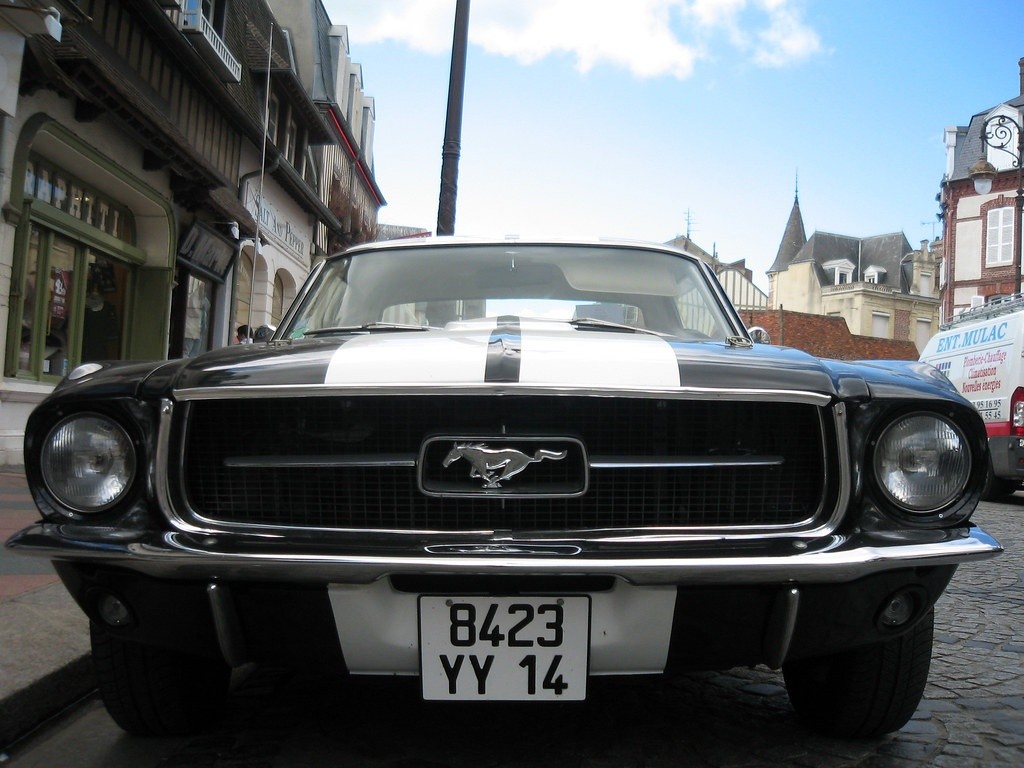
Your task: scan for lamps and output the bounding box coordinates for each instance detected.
[172,174,195,207]
[0,3,63,43]
[204,220,241,240]
[144,141,171,171]
[75,100,106,122]
[936,211,945,222]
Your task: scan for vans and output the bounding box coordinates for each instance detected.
[917,310,1024,500]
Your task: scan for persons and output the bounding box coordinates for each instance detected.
[235,325,254,346]
[182,282,211,357]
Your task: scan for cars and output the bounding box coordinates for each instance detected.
[3,232,1005,738]
